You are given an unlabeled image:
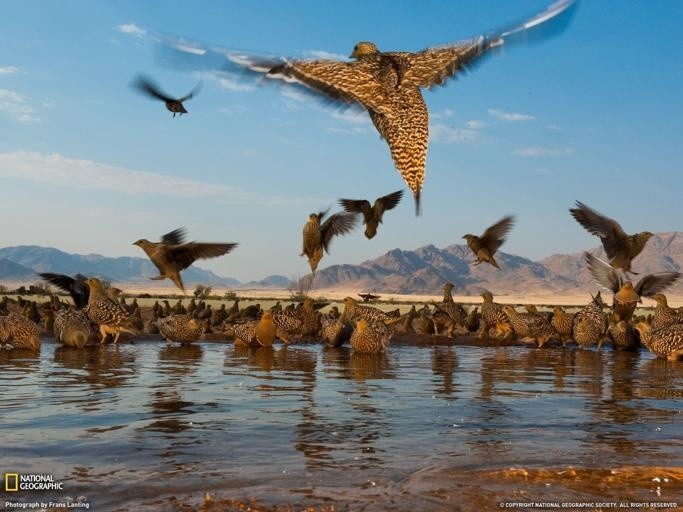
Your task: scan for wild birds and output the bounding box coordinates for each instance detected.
[297,188,403,271]
[567,199,655,280]
[132,223,238,297]
[174,0,576,216]
[137,77,194,118]
[460,213,522,271]
[1,250,683,360]
